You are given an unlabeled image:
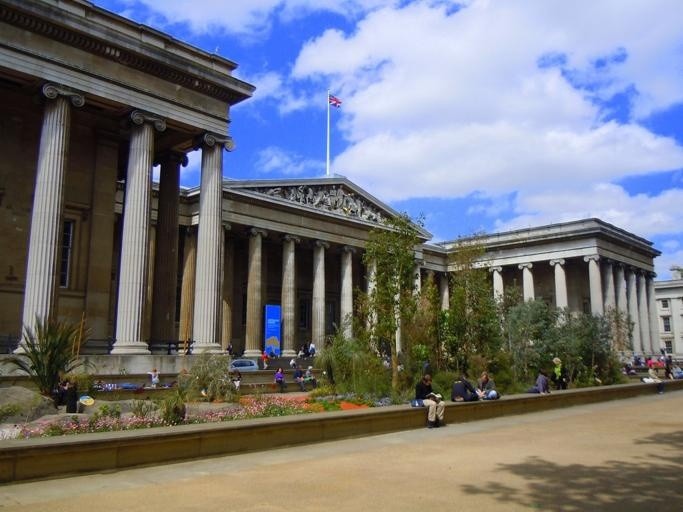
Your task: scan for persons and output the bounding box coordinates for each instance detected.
[527,369,552,395]
[415,374,447,428]
[451,375,479,402]
[51,340,320,407]
[551,357,570,390]
[621,352,683,394]
[476,371,501,400]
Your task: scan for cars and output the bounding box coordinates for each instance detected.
[230,359,258,371]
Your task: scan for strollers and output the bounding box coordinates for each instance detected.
[669,364,682,379]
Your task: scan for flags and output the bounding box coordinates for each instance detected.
[329,94,342,108]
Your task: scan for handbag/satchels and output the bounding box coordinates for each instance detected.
[411,399,426,407]
